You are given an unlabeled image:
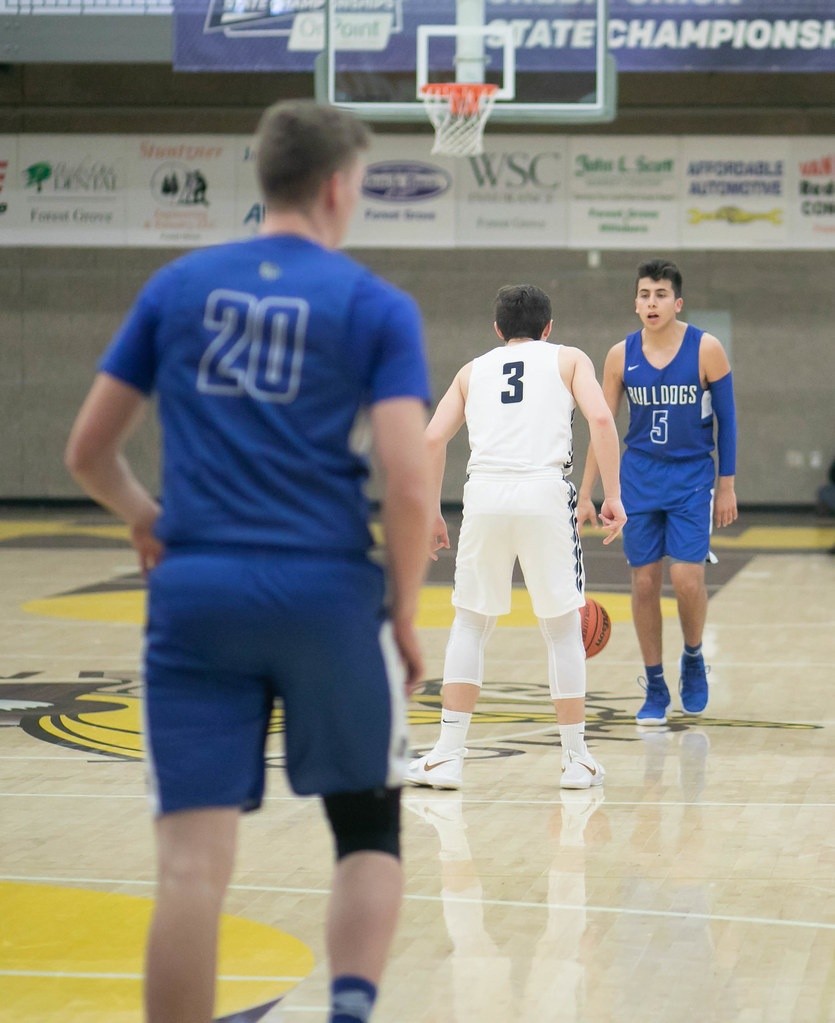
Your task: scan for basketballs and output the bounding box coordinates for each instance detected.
[578,597,610,659]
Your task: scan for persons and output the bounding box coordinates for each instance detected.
[403,285,628,791]
[578,257,738,727]
[65,103,427,1023]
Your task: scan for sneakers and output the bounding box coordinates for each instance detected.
[678,659,711,714]
[559,749,606,789]
[404,747,467,789]
[402,794,462,823]
[636,726,677,758]
[559,789,606,825]
[635,675,673,725]
[679,731,709,799]
